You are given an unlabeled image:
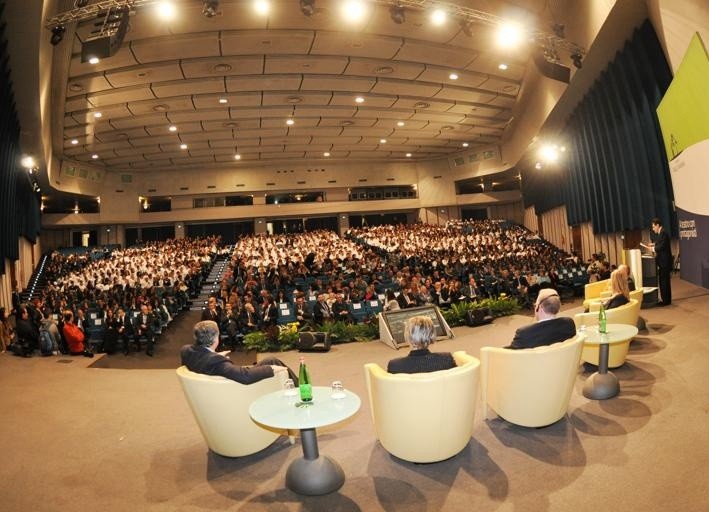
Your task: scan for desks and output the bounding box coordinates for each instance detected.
[249,383,361,497]
[578,321,638,399]
[624,246,661,311]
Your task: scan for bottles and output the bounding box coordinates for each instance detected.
[299,357,312,403]
[599,302,606,333]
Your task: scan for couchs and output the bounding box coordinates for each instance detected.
[364,350,479,465]
[574,296,639,372]
[175,349,291,456]
[585,275,618,313]
[584,285,644,332]
[480,328,587,426]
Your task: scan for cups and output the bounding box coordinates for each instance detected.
[330,381,344,400]
[283,379,297,397]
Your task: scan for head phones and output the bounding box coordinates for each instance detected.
[535,294,560,312]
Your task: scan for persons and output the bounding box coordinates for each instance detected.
[10,286,23,311]
[14,305,39,355]
[483,279,500,301]
[617,264,637,293]
[157,304,179,335]
[108,282,189,304]
[566,250,581,264]
[449,279,473,304]
[501,287,576,350]
[313,293,335,328]
[102,309,116,325]
[387,315,459,375]
[258,295,280,331]
[536,269,553,288]
[595,262,611,281]
[37,307,63,357]
[382,287,400,312]
[74,310,91,349]
[106,307,135,356]
[459,256,510,279]
[232,229,375,254]
[363,256,416,288]
[40,244,114,282]
[217,255,362,302]
[510,266,519,280]
[363,288,379,302]
[221,303,241,353]
[180,320,300,388]
[0,305,17,356]
[416,256,459,284]
[397,287,418,308]
[293,297,315,330]
[61,310,88,356]
[133,305,155,357]
[520,264,531,275]
[502,279,510,287]
[525,271,541,299]
[114,234,230,281]
[463,277,483,305]
[548,267,563,290]
[549,255,567,267]
[332,293,358,326]
[416,284,434,306]
[587,253,603,283]
[37,282,107,317]
[582,269,630,314]
[343,218,565,255]
[200,301,222,348]
[430,281,453,309]
[510,256,548,263]
[510,270,533,306]
[238,303,262,336]
[647,218,674,307]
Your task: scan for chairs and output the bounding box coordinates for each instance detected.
[91,251,104,260]
[352,302,366,320]
[554,269,559,275]
[91,318,105,339]
[295,277,305,286]
[58,243,87,256]
[576,270,588,291]
[571,268,581,276]
[107,243,120,250]
[151,318,162,332]
[378,293,386,301]
[158,311,168,323]
[133,310,142,318]
[89,312,98,321]
[276,302,292,311]
[394,291,402,297]
[556,279,570,297]
[128,317,135,325]
[306,276,315,283]
[581,267,588,275]
[367,299,383,314]
[308,295,317,304]
[567,272,583,295]
[561,269,569,276]
[278,308,295,322]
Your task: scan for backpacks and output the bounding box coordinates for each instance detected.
[38,321,54,358]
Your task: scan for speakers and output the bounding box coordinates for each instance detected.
[81,5,130,63]
[467,307,493,327]
[298,332,330,351]
